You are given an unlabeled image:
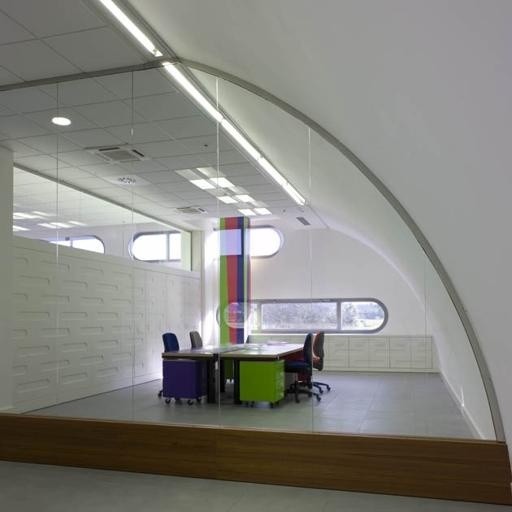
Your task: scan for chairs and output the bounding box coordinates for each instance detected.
[284,333,322,403]
[304,331,332,394]
[191,331,203,349]
[158,333,202,405]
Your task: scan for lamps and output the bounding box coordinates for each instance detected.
[82,0,308,208]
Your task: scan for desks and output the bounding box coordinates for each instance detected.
[219,343,305,407]
[162,342,258,404]
